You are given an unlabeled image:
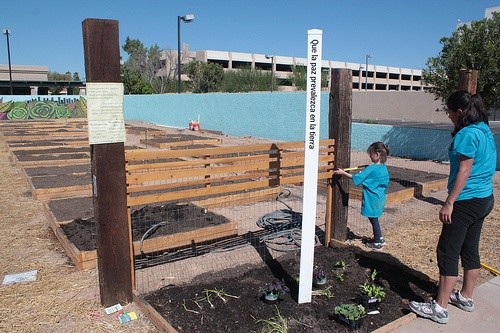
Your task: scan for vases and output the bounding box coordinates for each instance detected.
[264,295,280,305]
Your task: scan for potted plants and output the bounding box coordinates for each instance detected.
[356,269,387,309]
[334,304,367,331]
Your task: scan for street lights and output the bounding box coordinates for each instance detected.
[364,53,375,90]
[177,13,195,93]
[3,28,13,95]
[264,53,274,92]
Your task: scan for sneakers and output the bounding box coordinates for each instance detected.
[449,290,475,312]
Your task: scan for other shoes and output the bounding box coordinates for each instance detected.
[370,239,385,245]
[367,243,381,250]
[409,301,449,323]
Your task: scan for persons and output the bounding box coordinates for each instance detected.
[333,141,390,246]
[408,90,496,324]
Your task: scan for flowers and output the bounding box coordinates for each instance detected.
[258,278,290,299]
